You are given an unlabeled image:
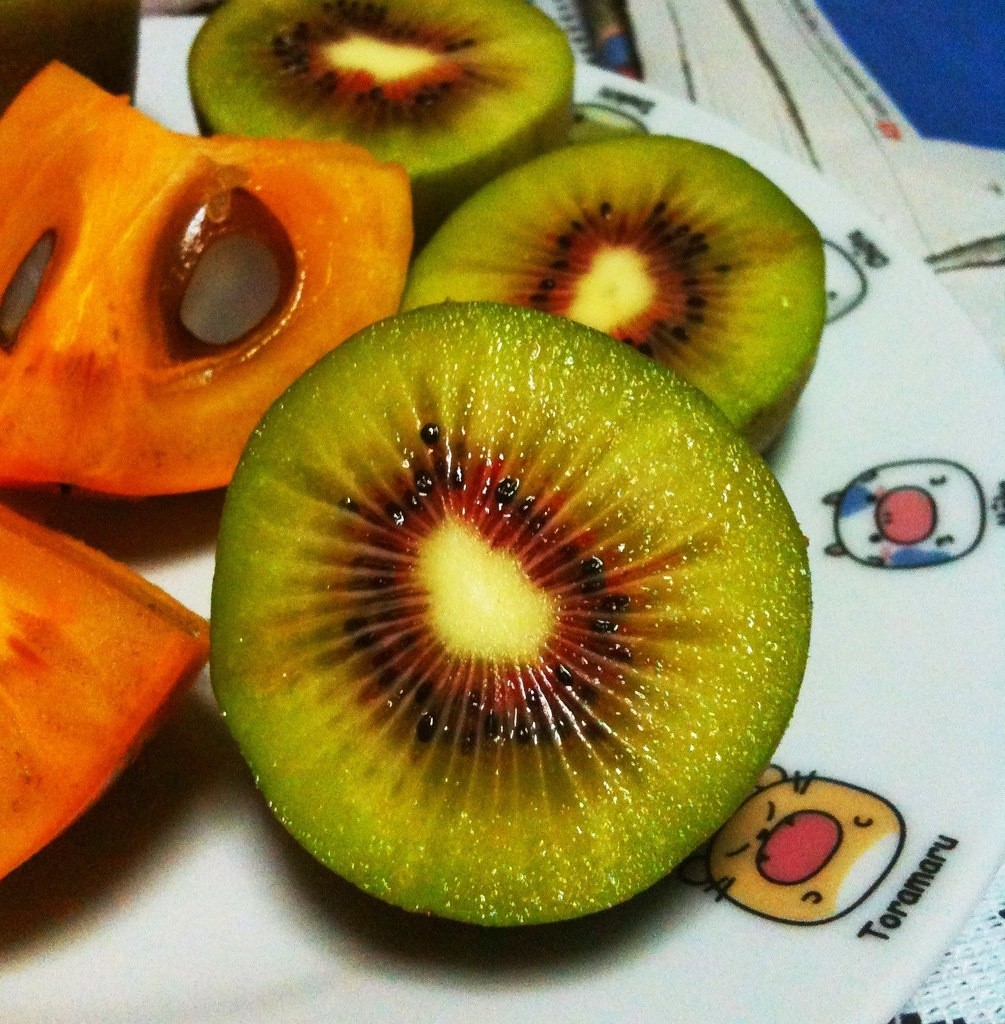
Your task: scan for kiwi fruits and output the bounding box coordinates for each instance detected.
[186,1,575,228]
[209,301,812,927]
[404,136,824,448]
